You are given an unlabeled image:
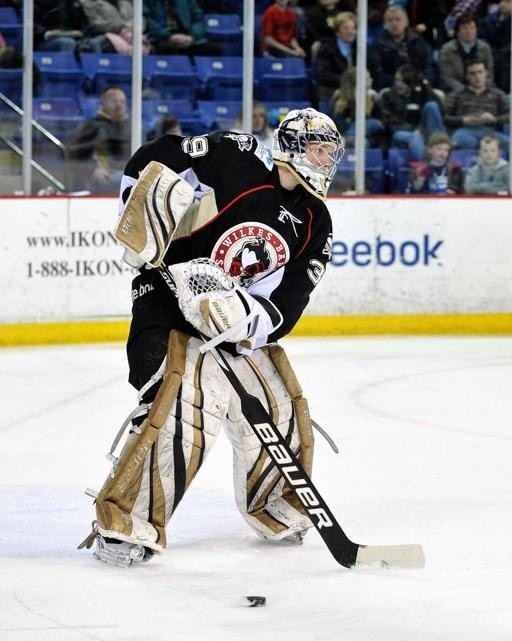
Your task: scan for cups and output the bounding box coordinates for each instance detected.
[436,176,448,196]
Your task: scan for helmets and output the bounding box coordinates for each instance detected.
[269,107,348,202]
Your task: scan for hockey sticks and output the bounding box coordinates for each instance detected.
[200,334,427,569]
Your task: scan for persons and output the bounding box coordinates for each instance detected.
[74,104,347,565]
[0,0,512,198]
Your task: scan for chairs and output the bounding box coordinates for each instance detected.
[0,0,512,198]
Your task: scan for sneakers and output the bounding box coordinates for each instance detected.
[293,526,309,537]
[93,526,154,564]
